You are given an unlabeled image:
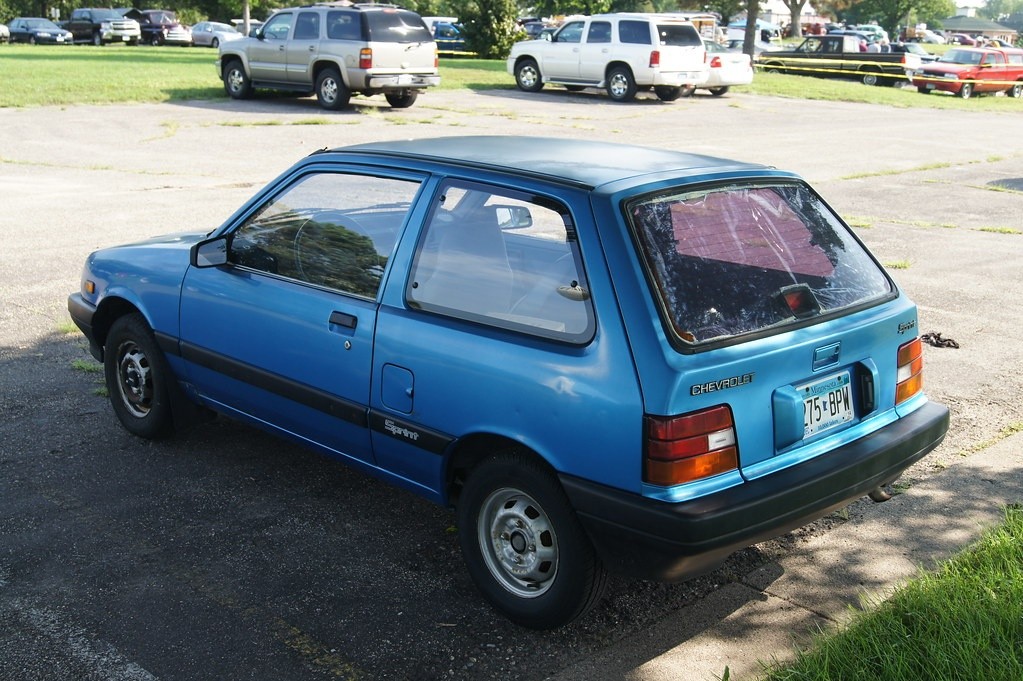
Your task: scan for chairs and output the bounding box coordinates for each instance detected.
[508,252,575,325]
[417,221,513,318]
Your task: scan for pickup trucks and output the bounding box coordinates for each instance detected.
[757,35,922,87]
[913,45,1023,99]
[57,8,141,46]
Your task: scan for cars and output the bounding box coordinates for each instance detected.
[190,21,245,47]
[66,134,951,634]
[0,24,10,45]
[696,37,753,96]
[717,36,783,60]
[523,22,551,40]
[230,19,264,38]
[818,23,1015,55]
[134,10,193,47]
[8,18,73,46]
[421,15,465,40]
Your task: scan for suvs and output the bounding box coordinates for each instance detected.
[505,13,706,102]
[217,6,440,109]
